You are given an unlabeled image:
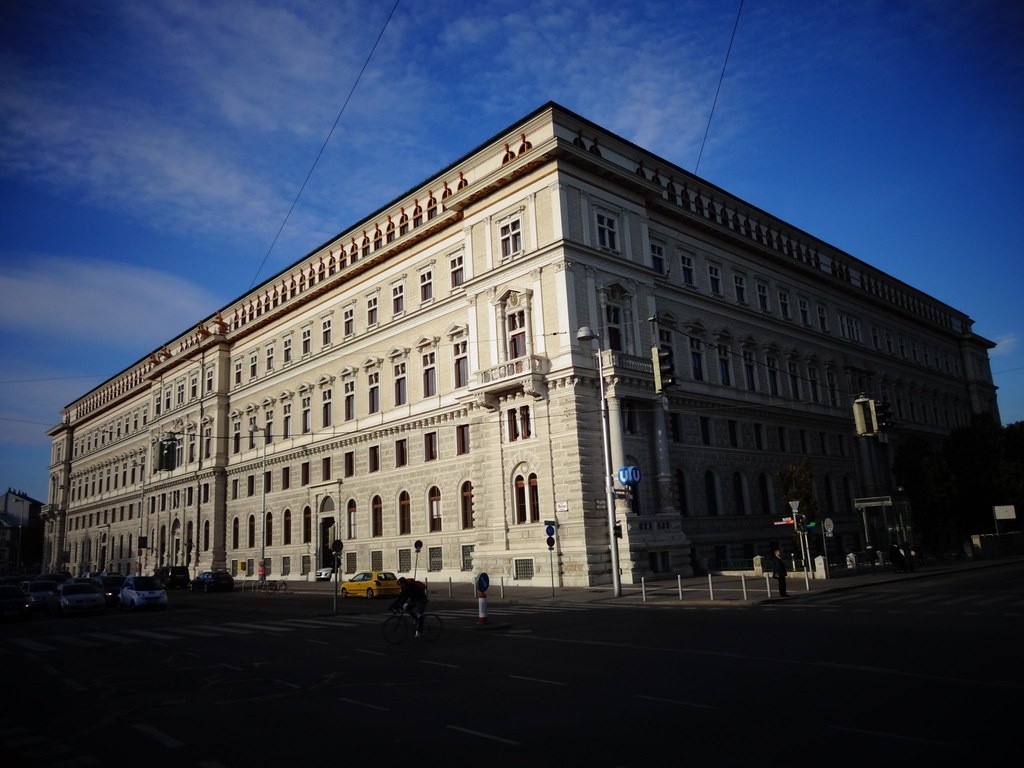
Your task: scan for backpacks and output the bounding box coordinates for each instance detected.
[408,579,425,593]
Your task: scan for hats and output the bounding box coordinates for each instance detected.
[396,577,407,585]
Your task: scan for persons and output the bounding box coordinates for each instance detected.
[154,568,168,584]
[890,543,906,573]
[773,548,791,598]
[982,535,993,558]
[390,577,429,638]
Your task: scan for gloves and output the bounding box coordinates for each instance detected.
[387,605,394,611]
[394,604,401,610]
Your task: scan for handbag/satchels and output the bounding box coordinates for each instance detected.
[772,572,782,579]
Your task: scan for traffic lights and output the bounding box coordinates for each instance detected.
[869,399,896,434]
[165,442,176,471]
[651,347,677,394]
[613,524,622,538]
[806,511,815,531]
[847,403,866,440]
[152,442,164,474]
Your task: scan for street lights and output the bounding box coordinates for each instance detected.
[577,327,622,597]
[248,424,266,581]
[58,485,70,572]
[12,498,24,568]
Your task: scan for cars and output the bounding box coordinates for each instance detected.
[340,572,402,601]
[0,566,189,623]
[44,583,105,620]
[316,566,334,578]
[188,572,234,593]
[116,576,168,613]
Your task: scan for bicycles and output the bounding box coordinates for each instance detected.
[382,607,443,645]
[261,579,288,594]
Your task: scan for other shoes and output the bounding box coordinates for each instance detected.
[415,632,424,639]
